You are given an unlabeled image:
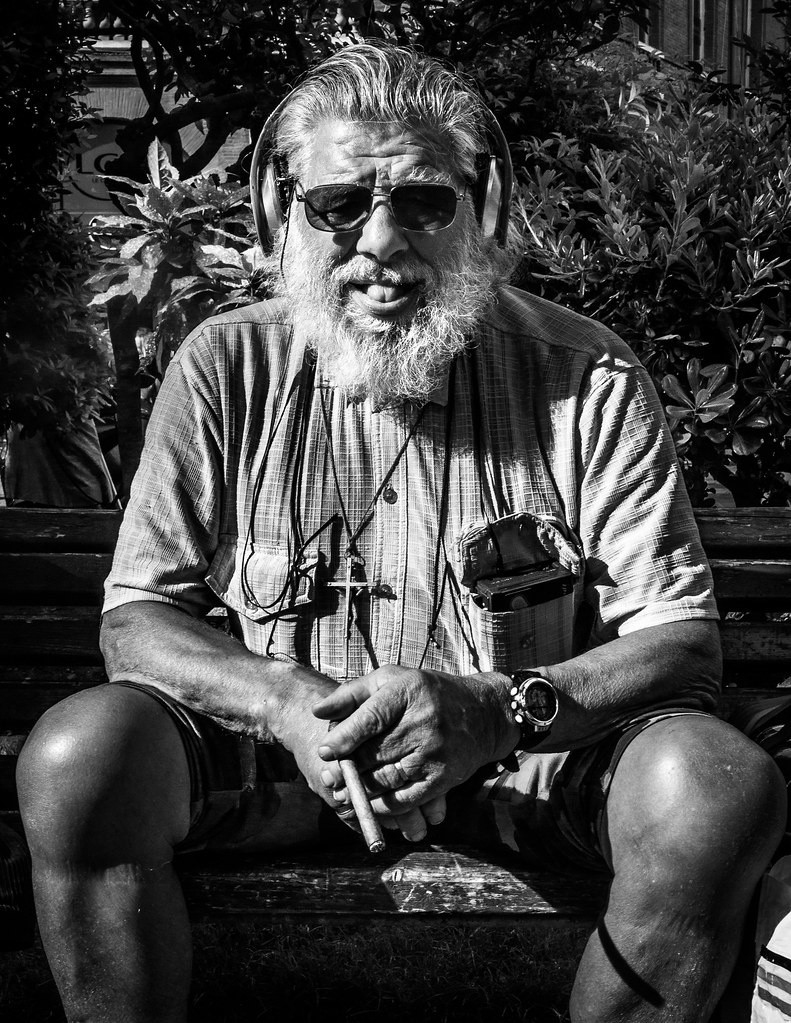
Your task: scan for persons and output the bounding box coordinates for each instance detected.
[14,37,790,1023]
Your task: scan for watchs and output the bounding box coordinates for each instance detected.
[498,668,558,752]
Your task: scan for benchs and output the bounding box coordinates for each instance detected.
[1,506,791,920]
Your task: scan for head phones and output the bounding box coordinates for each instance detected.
[249,82,514,258]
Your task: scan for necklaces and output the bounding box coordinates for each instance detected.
[319,371,424,638]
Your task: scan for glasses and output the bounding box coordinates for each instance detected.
[291,176,469,235]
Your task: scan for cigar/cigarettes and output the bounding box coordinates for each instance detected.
[328,722,387,853]
